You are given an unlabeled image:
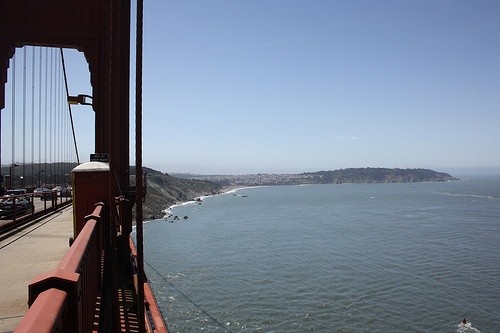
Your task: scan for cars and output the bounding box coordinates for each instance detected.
[41,189,57,202]
[34,186,73,197]
[3,189,27,197]
[0,195,35,220]
